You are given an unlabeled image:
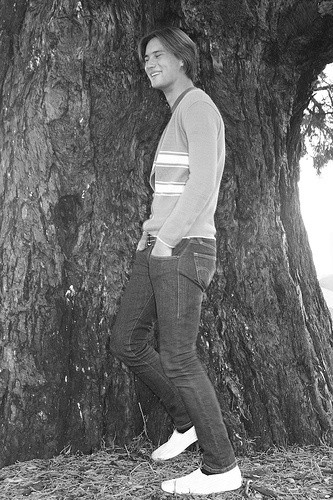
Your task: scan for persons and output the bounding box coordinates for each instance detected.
[108,25,242,495]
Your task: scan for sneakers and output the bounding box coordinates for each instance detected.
[152,426,198,461]
[161,465,243,495]
